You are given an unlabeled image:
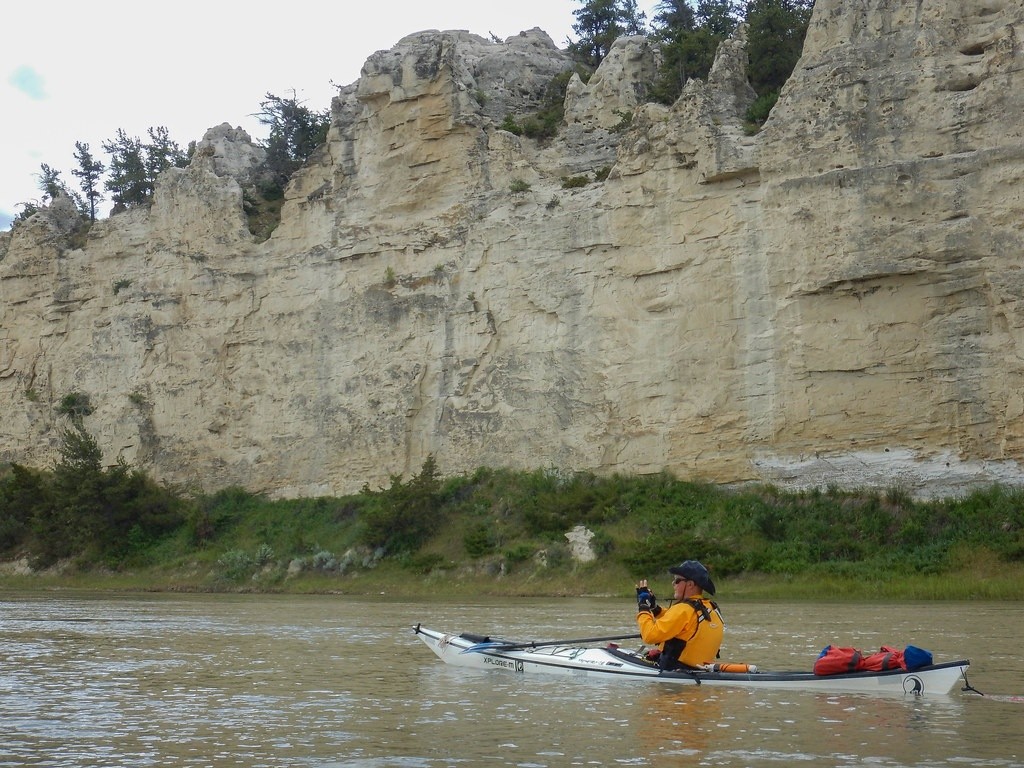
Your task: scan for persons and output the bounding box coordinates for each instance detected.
[635,560,724,668]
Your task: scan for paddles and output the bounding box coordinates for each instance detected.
[460,634,644,653]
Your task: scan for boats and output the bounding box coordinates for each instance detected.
[411,624,972,697]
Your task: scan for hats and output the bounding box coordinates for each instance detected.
[669,560,715,595]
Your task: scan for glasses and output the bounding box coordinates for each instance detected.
[674,578,689,585]
